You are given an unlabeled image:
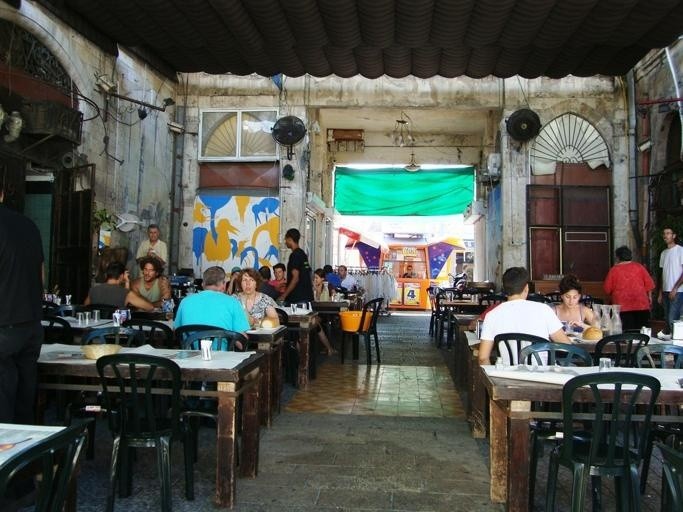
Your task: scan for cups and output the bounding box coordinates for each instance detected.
[65,294,72,306]
[43,292,52,301]
[291,303,297,314]
[83,312,92,325]
[675,376,683,385]
[598,358,611,373]
[91,310,100,323]
[172,287,194,299]
[331,294,344,302]
[53,296,61,306]
[76,312,85,326]
[111,312,120,327]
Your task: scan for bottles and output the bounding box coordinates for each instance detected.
[199,340,212,361]
[319,281,328,302]
[591,304,623,336]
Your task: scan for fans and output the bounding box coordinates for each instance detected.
[504,108,543,153]
[269,115,306,160]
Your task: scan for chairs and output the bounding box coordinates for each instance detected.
[494,331,682,512]
[2,416,94,512]
[36,286,348,467]
[427,285,603,349]
[340,297,384,364]
[97,355,201,511]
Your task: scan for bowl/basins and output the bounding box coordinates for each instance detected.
[338,310,372,333]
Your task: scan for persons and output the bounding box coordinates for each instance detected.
[0,180,48,425]
[601,244,658,333]
[224,263,361,313]
[133,221,171,278]
[477,264,572,365]
[227,267,281,329]
[118,255,173,309]
[467,302,504,331]
[548,272,600,332]
[401,264,417,278]
[170,265,253,352]
[75,260,156,316]
[276,227,339,357]
[653,227,683,330]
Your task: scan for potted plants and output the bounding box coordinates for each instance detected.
[90,200,117,249]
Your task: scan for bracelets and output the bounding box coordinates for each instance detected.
[281,294,286,299]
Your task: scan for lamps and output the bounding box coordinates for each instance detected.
[403,143,421,172]
[384,110,416,149]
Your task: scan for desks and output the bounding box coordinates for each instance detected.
[37,339,266,510]
[41,313,288,429]
[481,365,683,512]
[278,308,320,390]
[440,298,494,351]
[463,332,674,439]
[277,285,367,365]
[452,314,481,388]
